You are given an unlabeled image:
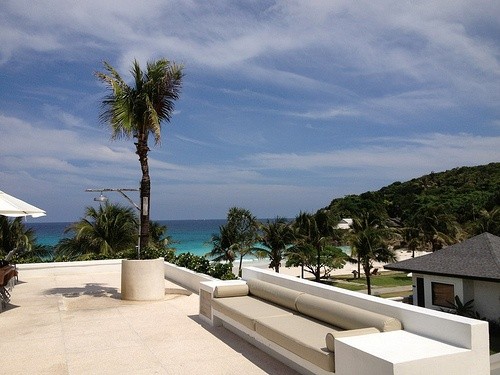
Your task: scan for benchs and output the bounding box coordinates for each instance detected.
[196,265,491,374]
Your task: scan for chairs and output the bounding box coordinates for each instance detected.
[0,259,19,309]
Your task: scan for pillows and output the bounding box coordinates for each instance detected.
[325,326,381,352]
[214,284,250,298]
[248,277,305,312]
[295,292,401,333]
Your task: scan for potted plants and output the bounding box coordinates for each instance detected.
[121,246,169,302]
